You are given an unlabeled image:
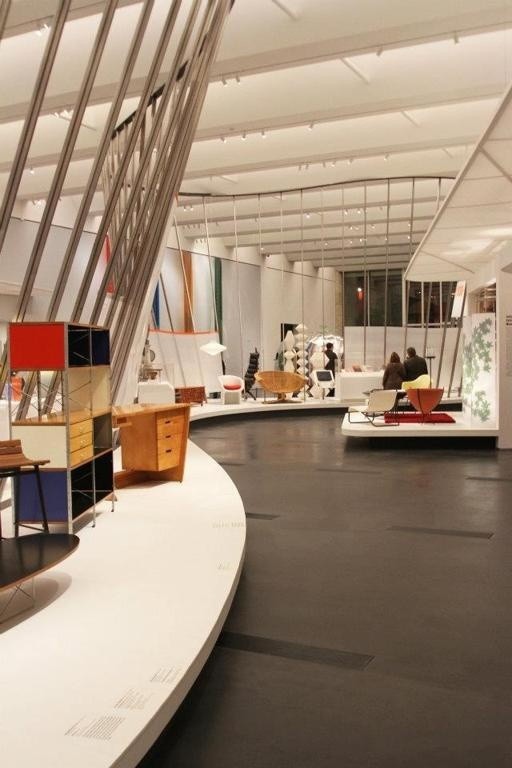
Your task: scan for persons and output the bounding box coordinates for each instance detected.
[404,347,428,381]
[382,352,404,390]
[324,343,338,397]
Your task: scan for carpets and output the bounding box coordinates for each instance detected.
[384,413,455,423]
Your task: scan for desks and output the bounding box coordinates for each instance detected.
[1,534,80,624]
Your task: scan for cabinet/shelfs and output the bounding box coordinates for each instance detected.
[7,320,117,535]
[113,401,193,486]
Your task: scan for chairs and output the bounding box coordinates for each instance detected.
[254,371,307,403]
[218,374,244,404]
[1,439,51,539]
[347,387,399,428]
[402,374,430,389]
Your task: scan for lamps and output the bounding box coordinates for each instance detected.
[181,75,390,258]
